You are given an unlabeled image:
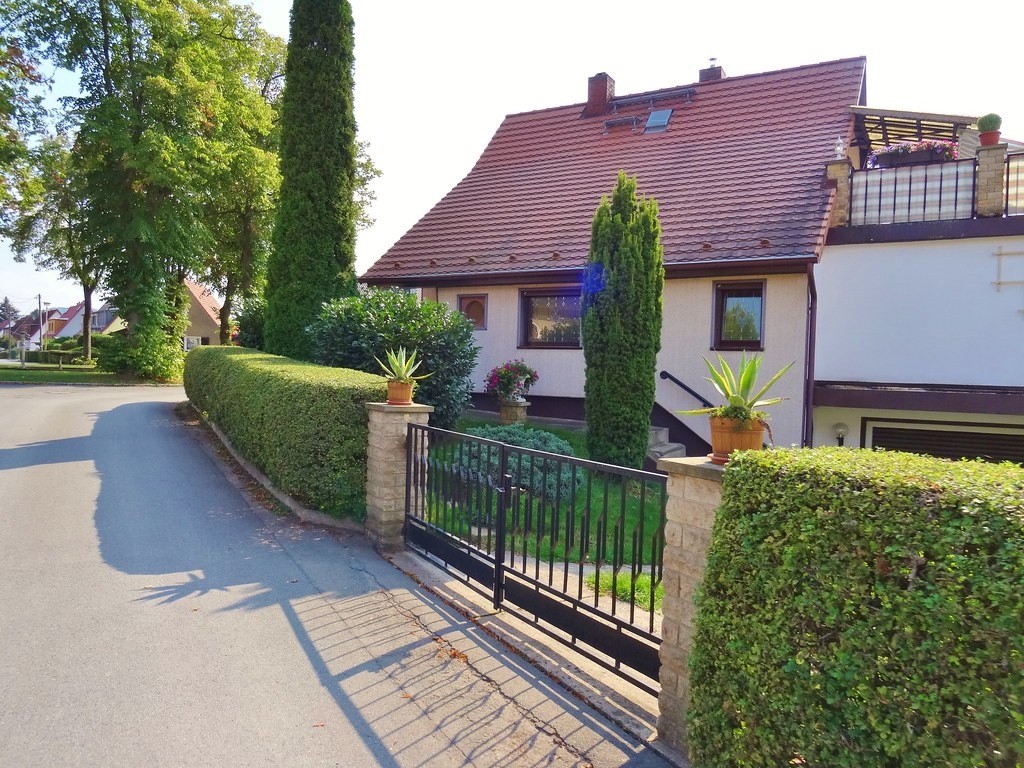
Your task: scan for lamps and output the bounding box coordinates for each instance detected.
[833,423,852,446]
[832,136,846,160]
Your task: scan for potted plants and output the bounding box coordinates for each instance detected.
[670,346,796,465]
[976,113,1003,144]
[373,345,438,405]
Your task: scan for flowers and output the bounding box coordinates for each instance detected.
[868,137,959,168]
[480,358,539,400]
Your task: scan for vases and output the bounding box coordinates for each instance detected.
[877,147,948,168]
[497,400,533,427]
